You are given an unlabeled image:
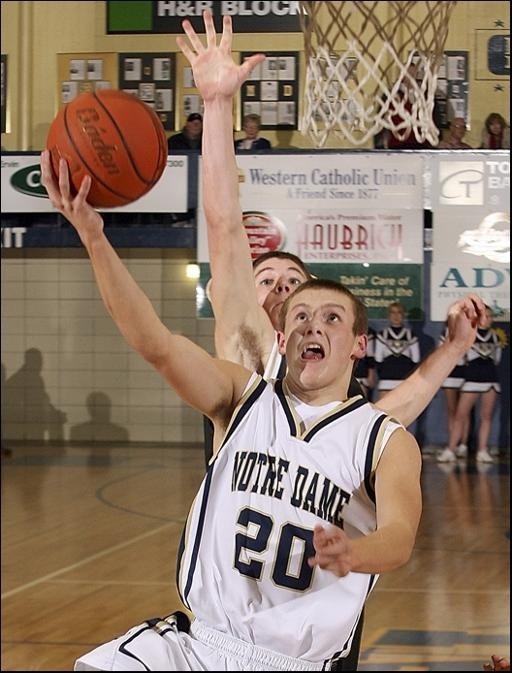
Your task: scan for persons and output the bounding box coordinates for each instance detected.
[371,61,510,150]
[168,113,272,151]
[38,146,424,672]
[352,302,506,463]
[176,6,491,673]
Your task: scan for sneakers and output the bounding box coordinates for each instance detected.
[436,444,469,462]
[476,451,496,463]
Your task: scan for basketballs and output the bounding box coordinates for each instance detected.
[46,88,166,207]
